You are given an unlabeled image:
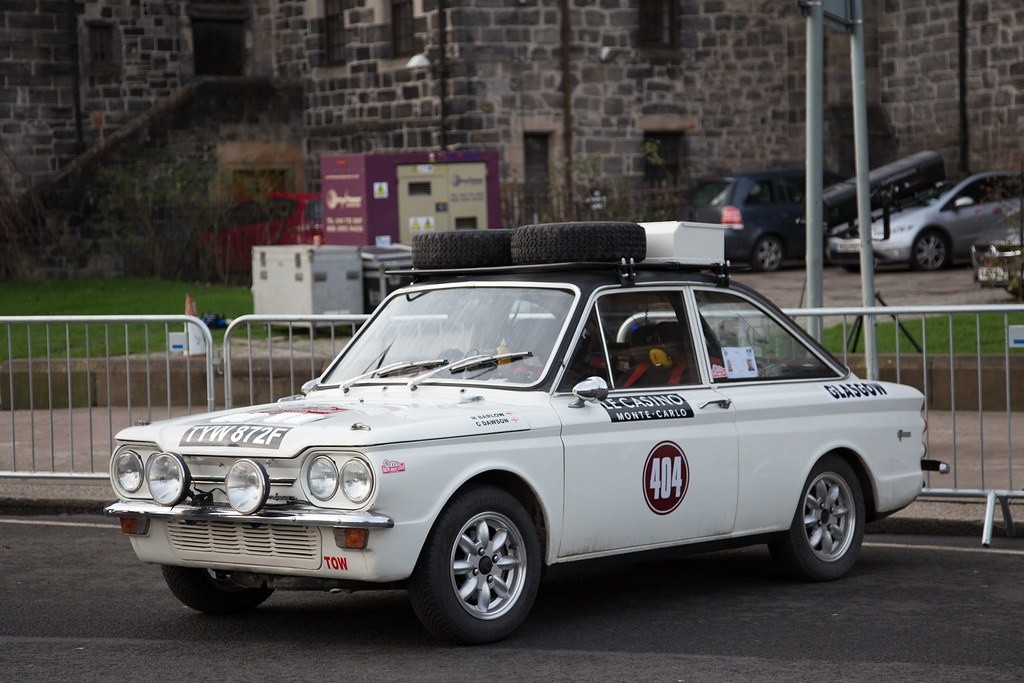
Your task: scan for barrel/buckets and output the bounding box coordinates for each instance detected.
[169,332,186,352]
[1009,325,1024,348]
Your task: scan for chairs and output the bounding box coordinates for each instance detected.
[616,322,688,390]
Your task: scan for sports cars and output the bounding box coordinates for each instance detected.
[105,211,930,642]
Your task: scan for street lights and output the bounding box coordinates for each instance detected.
[406,53,448,153]
[563,40,623,220]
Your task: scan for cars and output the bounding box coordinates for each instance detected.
[188,193,323,281]
[825,168,1022,271]
[664,162,862,273]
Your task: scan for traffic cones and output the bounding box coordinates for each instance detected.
[180,292,207,357]
